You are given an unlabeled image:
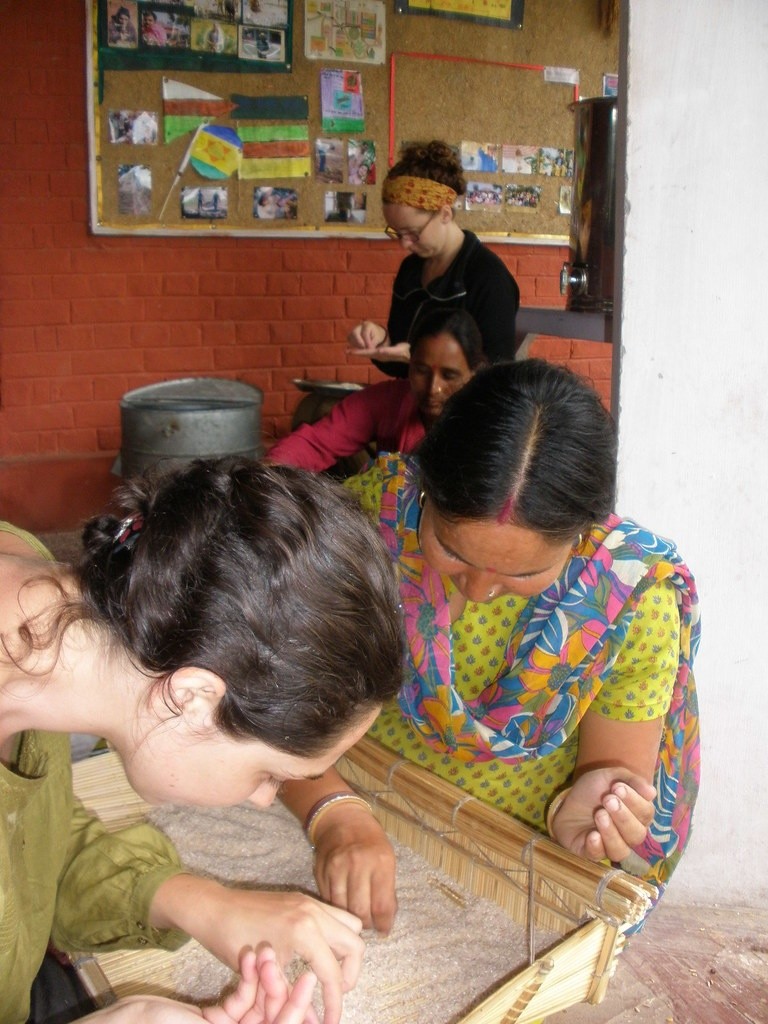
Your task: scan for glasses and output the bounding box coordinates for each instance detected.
[383,209,436,244]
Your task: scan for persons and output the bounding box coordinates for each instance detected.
[143,12,190,49]
[0,455,405,1024]
[110,5,135,47]
[255,33,270,58]
[203,23,225,54]
[344,139,519,378]
[276,359,703,952]
[258,307,492,480]
[122,114,577,223]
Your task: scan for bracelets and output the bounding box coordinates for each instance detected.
[376,322,388,348]
[547,785,574,841]
[305,791,375,851]
